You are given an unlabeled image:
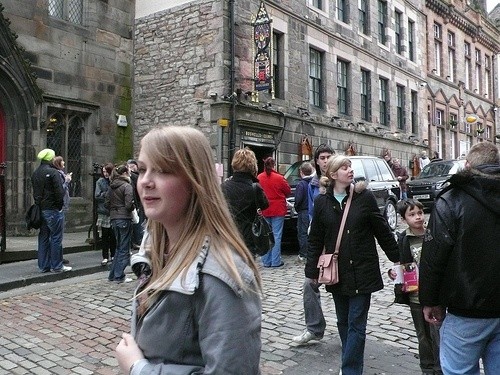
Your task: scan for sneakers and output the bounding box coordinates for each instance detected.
[292,330,320,343]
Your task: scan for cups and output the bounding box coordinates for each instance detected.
[393,265,404,284]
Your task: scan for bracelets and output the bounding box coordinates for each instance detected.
[128,360,140,375]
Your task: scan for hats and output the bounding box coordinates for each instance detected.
[38,149,55,161]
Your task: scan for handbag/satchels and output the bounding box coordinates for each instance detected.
[130,208,139,224]
[24,204,41,231]
[317,254,339,285]
[250,214,275,256]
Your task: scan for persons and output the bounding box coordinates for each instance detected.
[305,155,400,375]
[418,141,500,375]
[32,149,148,283]
[388,199,446,375]
[116,125,266,375]
[219,147,440,345]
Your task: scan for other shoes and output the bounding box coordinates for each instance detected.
[115,278,133,283]
[51,265,72,272]
[295,256,305,262]
[101,259,108,266]
[273,261,284,267]
[62,259,69,264]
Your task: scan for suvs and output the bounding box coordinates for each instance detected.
[406,157,470,211]
[280,155,402,252]
